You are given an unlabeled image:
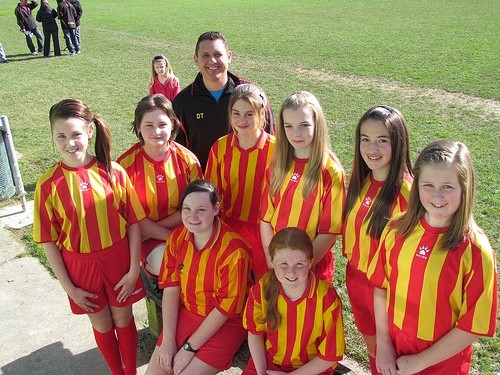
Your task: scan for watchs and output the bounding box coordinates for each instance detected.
[183,342,198,353]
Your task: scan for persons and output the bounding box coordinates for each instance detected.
[14,0,44,55]
[36,0,63,56]
[341,105,414,375]
[171,31,275,175]
[242,227,346,375]
[367,140,498,375]
[258,90,347,285]
[31,97,147,375]
[204,84,276,226]
[148,53,180,101]
[145,178,253,375]
[57,0,83,56]
[116,93,205,242]
